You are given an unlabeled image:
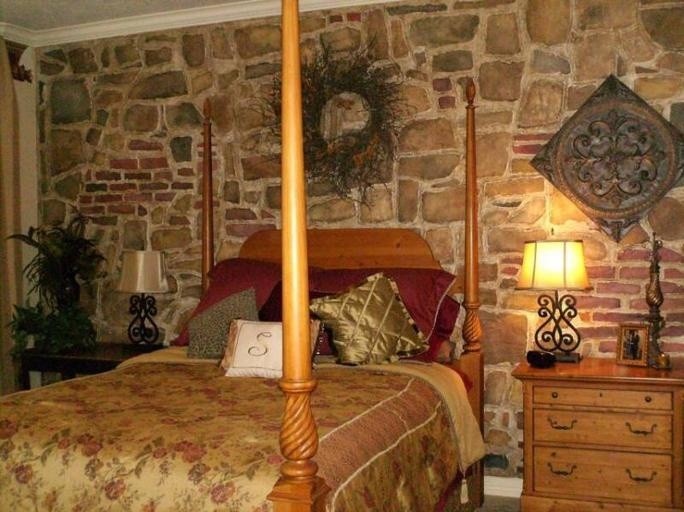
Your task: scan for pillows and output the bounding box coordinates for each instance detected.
[168,258,466,380]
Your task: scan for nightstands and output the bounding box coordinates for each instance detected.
[21,340,168,373]
[511,354,684,512]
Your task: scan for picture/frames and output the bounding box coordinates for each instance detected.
[615,321,650,367]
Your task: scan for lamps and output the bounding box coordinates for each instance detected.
[114,249,169,344]
[513,240,590,363]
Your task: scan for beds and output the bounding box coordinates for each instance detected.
[0,227,484,511]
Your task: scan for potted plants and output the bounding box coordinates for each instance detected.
[3,209,109,313]
